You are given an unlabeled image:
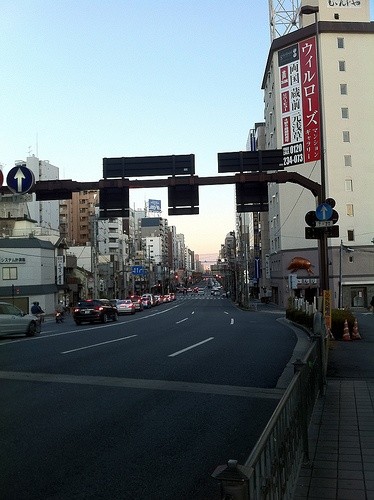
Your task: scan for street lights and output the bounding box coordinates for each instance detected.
[229,232,239,303]
[339,239,354,309]
[148,244,153,270]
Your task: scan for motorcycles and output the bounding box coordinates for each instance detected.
[55,305,68,324]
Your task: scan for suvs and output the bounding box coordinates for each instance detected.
[73,298,119,324]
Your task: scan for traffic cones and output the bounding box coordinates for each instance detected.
[351,318,362,340]
[339,317,353,341]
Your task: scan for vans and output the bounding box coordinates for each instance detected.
[142,293,156,308]
[198,287,205,295]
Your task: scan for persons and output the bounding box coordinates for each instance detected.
[56,300,66,320]
[30,301,44,313]
[293,293,305,310]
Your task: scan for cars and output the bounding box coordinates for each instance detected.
[109,295,152,315]
[0,302,41,337]
[154,293,176,305]
[186,287,193,292]
[211,286,224,296]
